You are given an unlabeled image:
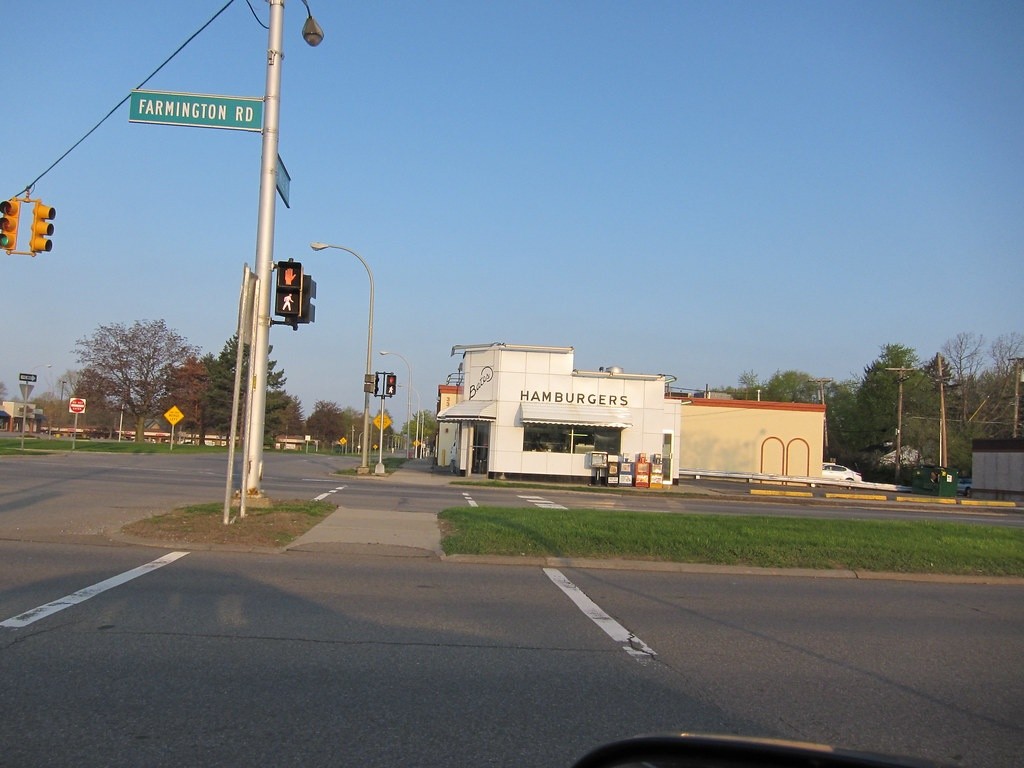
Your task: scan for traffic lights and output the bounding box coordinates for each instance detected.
[386,375,396,395]
[274,261,303,318]
[30,200,56,254]
[364,374,377,392]
[0,201,21,250]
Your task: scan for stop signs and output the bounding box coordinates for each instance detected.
[69,398,86,414]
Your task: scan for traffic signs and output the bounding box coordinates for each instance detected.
[128,88,263,134]
[19,374,36,382]
[277,152,292,210]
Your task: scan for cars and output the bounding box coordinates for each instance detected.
[823,464,862,482]
[957,476,972,497]
[82,426,117,440]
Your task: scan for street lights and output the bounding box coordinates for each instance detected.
[247,0,323,491]
[310,242,375,465]
[380,351,412,459]
[57,380,67,433]
[398,383,420,458]
